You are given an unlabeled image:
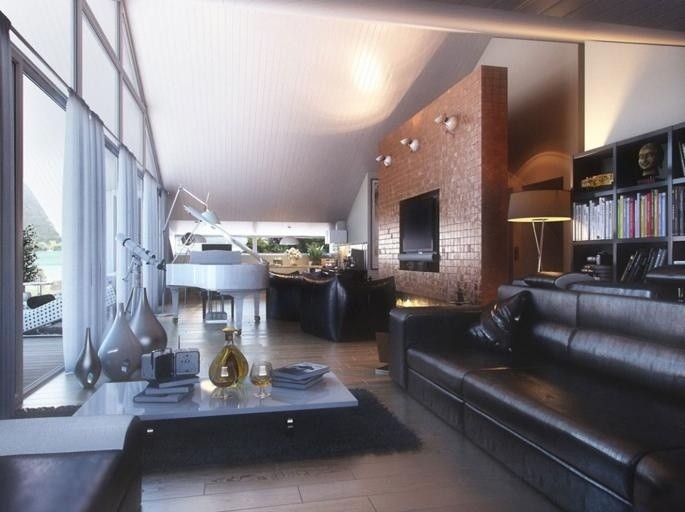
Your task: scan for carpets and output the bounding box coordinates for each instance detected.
[8,387,423,467]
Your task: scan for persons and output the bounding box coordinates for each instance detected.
[637,141,665,177]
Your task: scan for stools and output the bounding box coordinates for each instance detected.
[200,289,234,322]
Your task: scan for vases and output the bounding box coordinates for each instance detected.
[76,326,102,388]
[123,286,167,370]
[97,302,144,382]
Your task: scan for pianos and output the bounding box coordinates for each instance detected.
[165,203,269,335]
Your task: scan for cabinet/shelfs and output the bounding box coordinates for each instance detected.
[571,122,685,282]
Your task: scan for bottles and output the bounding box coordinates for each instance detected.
[209,327,249,388]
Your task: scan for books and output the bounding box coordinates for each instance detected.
[272,361,328,381]
[147,373,200,388]
[272,373,321,384]
[142,382,194,394]
[132,390,187,403]
[571,185,685,282]
[268,376,325,390]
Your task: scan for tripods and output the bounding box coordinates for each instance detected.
[124,290,148,316]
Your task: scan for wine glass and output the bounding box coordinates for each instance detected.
[216,361,238,401]
[250,360,273,399]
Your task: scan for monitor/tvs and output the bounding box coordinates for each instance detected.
[399,195,435,252]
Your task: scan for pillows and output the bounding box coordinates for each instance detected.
[452,290,530,353]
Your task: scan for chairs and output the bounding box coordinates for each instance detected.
[0,416,143,512]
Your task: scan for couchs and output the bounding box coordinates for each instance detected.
[386,277,685,512]
[268,271,394,342]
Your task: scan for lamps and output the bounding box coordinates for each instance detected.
[279,237,300,245]
[376,154,391,166]
[506,189,573,273]
[400,138,419,152]
[434,114,457,132]
[164,183,220,229]
